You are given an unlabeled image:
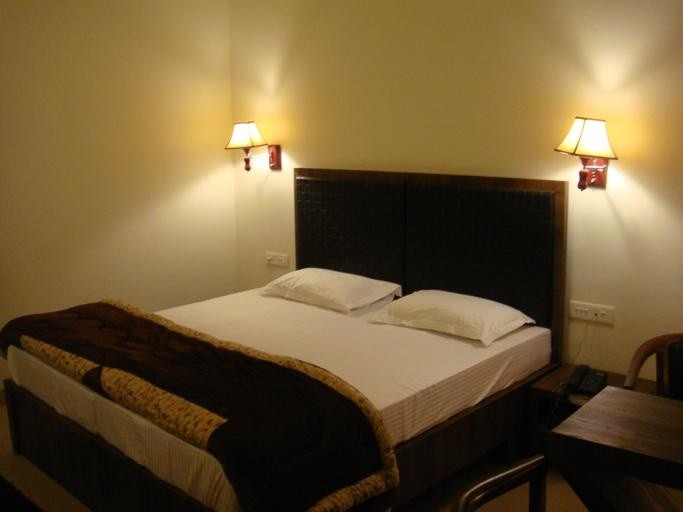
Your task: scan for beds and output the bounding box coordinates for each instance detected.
[2,169,581,512]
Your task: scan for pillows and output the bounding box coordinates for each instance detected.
[260,262,541,357]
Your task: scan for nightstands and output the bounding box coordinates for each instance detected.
[529,363,657,408]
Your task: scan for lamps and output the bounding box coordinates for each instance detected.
[224,120,269,171]
[553,114,619,190]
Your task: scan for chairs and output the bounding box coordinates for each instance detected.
[458,456,593,512]
[617,327,683,398]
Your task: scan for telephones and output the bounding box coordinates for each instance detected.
[561,364,608,397]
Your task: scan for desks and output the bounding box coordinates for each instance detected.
[544,375,683,484]
